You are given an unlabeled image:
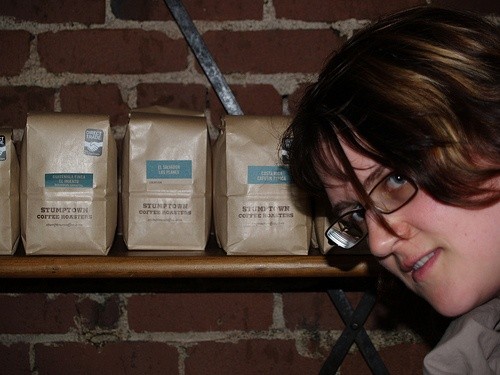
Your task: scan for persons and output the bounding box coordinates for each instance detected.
[276,1,499,375]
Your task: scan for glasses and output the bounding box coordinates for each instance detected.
[325,141,432,250]
